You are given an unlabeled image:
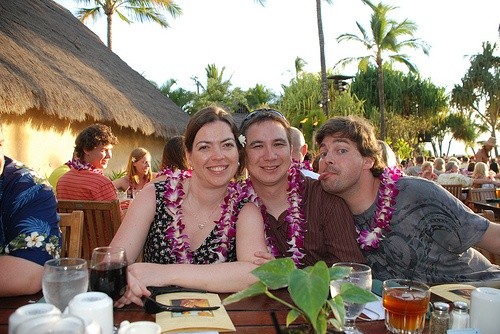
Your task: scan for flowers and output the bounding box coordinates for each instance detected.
[296,160,313,171]
[156,168,244,268]
[66,159,104,175]
[356,166,404,253]
[238,134,247,148]
[131,157,136,163]
[239,167,307,270]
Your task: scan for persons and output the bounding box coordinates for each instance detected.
[436,161,500,200]
[238,109,364,265]
[102,106,268,308]
[0,120,62,297]
[48,149,79,195]
[463,162,500,210]
[112,148,159,198]
[56,123,124,258]
[290,126,321,180]
[417,161,438,182]
[143,136,193,189]
[315,116,499,286]
[401,136,499,176]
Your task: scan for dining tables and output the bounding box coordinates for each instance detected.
[474,200,500,219]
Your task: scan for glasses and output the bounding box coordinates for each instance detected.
[144,287,220,314]
[245,110,283,119]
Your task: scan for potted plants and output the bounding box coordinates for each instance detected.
[222,257,380,334]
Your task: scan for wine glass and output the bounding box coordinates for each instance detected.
[117,192,127,202]
[88,247,128,334]
[330,262,372,334]
[42,258,89,313]
[133,190,141,199]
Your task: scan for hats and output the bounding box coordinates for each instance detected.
[482,137,498,147]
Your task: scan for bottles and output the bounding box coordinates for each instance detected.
[449,301,470,329]
[430,302,450,334]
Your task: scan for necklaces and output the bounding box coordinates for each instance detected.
[188,179,227,229]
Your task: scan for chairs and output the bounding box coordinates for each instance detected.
[55,198,119,268]
[442,184,462,202]
[471,188,496,202]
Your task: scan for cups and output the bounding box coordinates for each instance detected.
[9,291,161,334]
[382,279,431,334]
[470,287,500,334]
[496,187,500,199]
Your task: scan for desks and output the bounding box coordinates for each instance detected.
[0,290,454,334]
